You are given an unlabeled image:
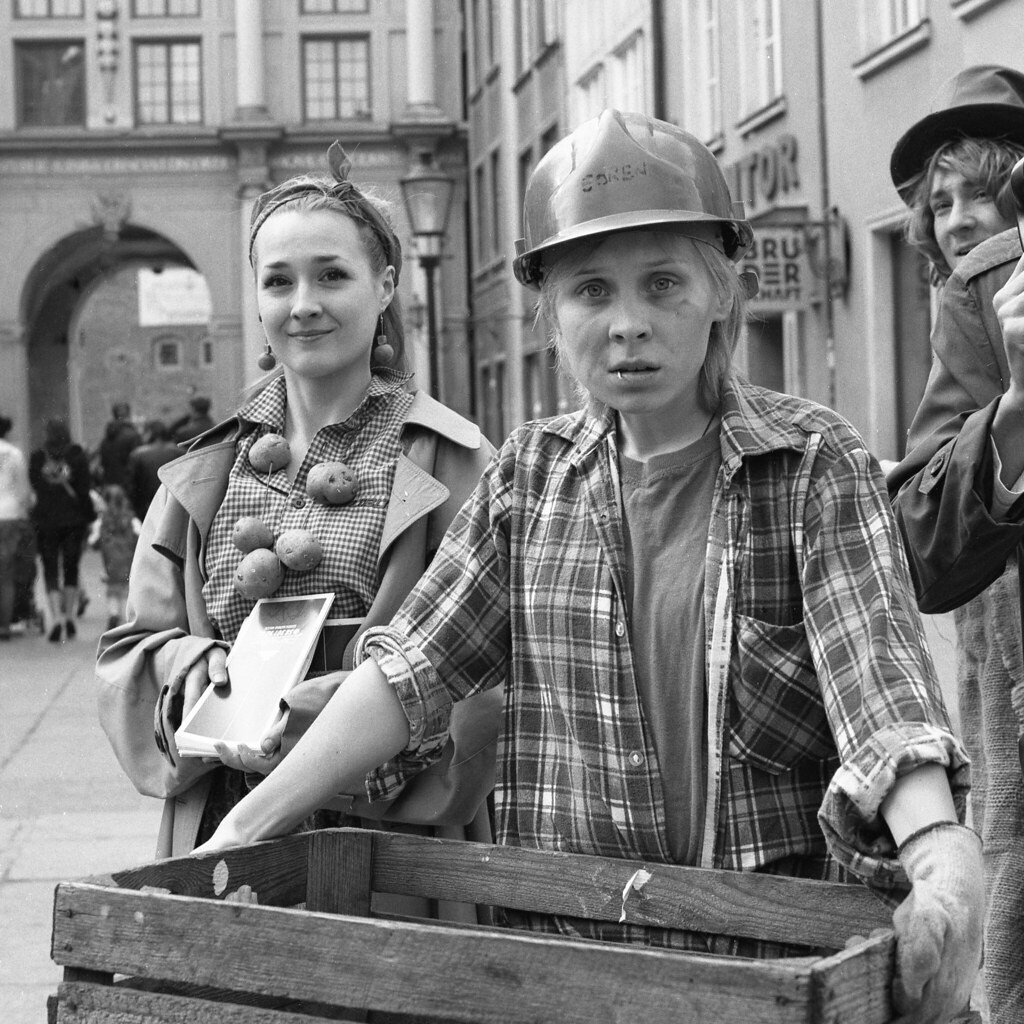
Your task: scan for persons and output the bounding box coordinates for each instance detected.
[885,64,1024,1023]
[98,396,219,521]
[94,140,501,924]
[185,109,991,1022]
[0,416,96,642]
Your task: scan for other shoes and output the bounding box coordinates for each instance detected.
[0,631,24,642]
[107,610,119,631]
[48,622,62,642]
[66,619,76,639]
[76,593,89,616]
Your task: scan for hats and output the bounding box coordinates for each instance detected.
[889,64,1024,212]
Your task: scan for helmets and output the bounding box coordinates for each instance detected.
[513,107,754,292]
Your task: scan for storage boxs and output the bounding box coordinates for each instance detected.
[46,827,912,1024]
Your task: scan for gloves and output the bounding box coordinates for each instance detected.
[844,820,985,1024]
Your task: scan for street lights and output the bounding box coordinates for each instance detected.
[398,149,458,403]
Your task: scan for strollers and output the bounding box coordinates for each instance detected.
[0,507,47,634]
